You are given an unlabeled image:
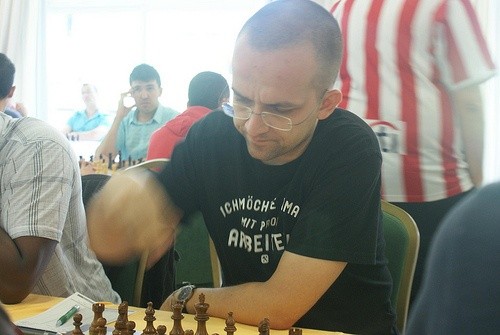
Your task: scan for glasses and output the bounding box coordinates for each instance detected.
[218,80,328,131]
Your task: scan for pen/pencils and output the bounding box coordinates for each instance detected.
[56,305,80,326]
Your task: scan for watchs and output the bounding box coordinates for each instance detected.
[176,285,197,314]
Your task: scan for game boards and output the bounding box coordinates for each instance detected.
[63,324,143,335]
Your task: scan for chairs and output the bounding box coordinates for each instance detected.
[380,201,421,335]
[79,158,213,307]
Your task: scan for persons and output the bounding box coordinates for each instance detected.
[95,63,180,162]
[326,0,497,324]
[61,83,115,155]
[0,52,30,119]
[86,0,398,335]
[0,110,122,305]
[146,71,230,175]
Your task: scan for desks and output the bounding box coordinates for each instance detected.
[79,157,144,174]
[0,293,351,335]
